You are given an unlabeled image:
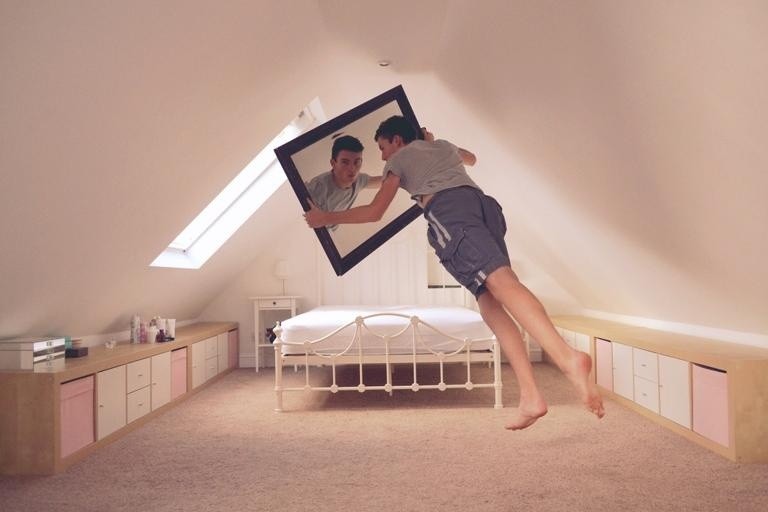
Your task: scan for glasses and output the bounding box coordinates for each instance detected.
[272,242,308,294]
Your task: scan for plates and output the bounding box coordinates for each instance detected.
[545,314,767,468]
[1,320,240,483]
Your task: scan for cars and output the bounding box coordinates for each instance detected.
[160,312,177,338]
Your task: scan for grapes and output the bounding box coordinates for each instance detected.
[463,285,543,364]
[248,294,301,373]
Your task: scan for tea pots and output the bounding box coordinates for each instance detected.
[272,84,430,275]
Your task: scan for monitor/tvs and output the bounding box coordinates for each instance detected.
[1,335,66,370]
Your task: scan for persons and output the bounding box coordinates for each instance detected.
[306,134,383,238]
[303,115,606,431]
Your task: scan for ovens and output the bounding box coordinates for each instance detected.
[271,304,505,410]
